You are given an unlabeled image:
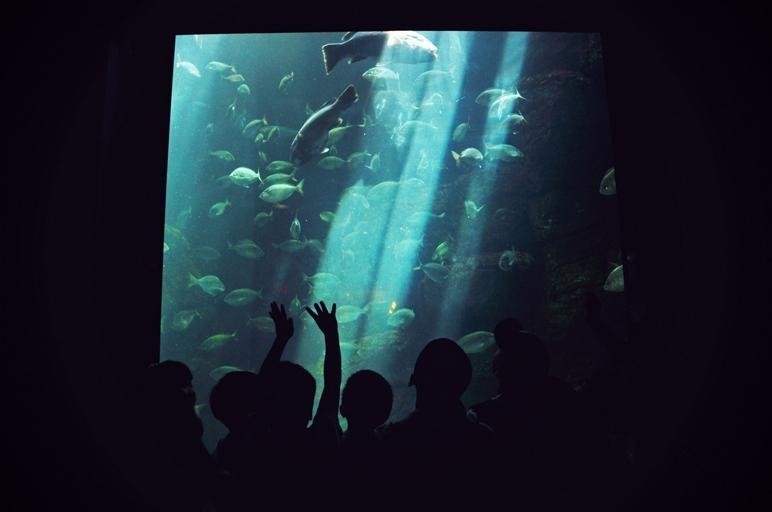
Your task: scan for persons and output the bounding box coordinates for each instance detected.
[132,302,393,510]
[375,333,636,511]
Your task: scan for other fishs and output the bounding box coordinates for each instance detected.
[161,56,530,427]
